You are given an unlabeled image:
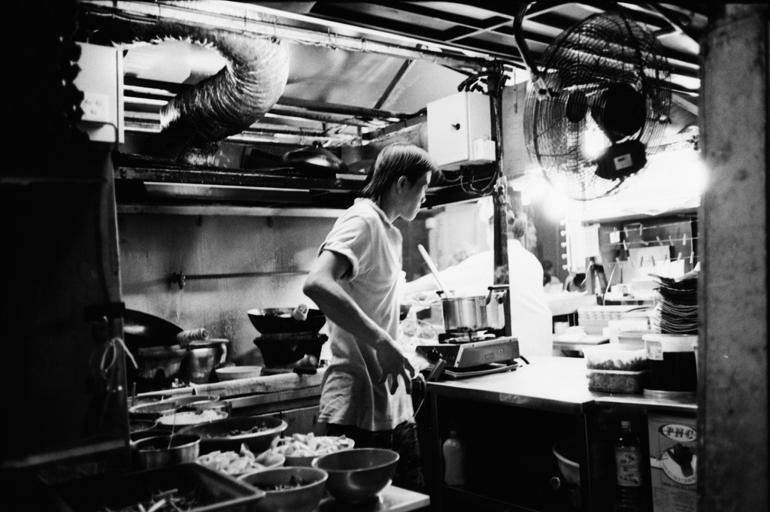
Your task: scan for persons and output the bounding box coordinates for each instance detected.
[301,142,440,489]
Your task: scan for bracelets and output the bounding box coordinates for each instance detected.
[372,330,393,354]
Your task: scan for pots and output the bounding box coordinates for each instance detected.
[435,281,510,342]
[246,302,328,333]
[132,431,199,468]
[131,337,230,392]
[123,306,209,347]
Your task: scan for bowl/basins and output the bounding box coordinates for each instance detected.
[212,363,262,382]
[274,434,355,466]
[234,467,328,510]
[311,448,402,504]
[179,415,288,454]
[130,392,228,435]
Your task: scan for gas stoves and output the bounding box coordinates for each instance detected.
[415,332,523,382]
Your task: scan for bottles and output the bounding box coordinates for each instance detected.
[443,430,466,487]
[613,420,643,510]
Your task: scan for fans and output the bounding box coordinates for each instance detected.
[522,12,662,202]
